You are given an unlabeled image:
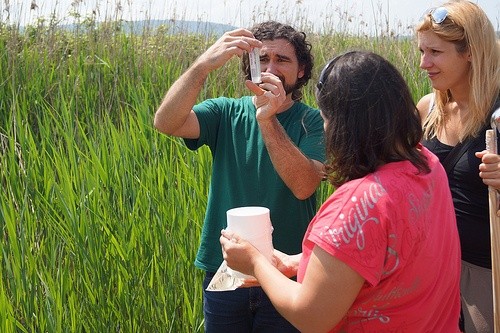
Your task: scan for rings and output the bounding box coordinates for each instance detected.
[275,93,281,98]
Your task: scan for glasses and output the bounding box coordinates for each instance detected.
[318,51,358,90]
[427,6,453,24]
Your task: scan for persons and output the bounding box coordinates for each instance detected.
[413,0,500,332]
[219,52,463,333]
[154,20,327,333]
